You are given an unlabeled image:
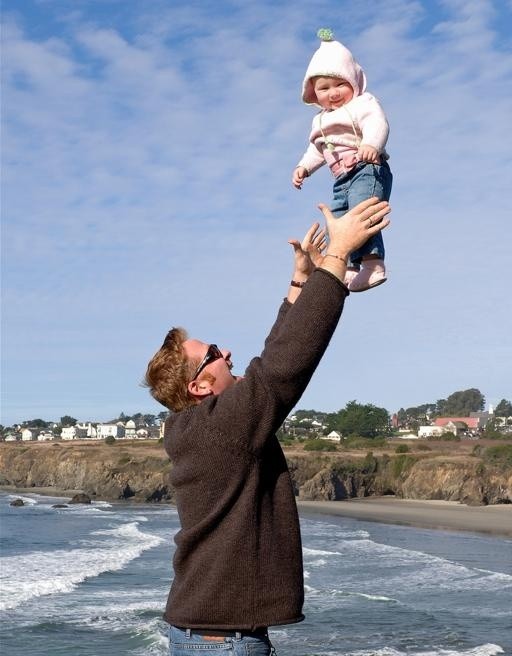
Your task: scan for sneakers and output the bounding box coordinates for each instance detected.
[345,258,388,292]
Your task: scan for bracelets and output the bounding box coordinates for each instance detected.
[290,279,307,289]
[322,250,348,267]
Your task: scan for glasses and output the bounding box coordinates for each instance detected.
[192,343,224,382]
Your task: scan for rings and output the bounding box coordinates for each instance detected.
[368,217,375,226]
[317,245,324,254]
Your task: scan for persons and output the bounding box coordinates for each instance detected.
[290,24,395,293]
[142,192,394,656]
